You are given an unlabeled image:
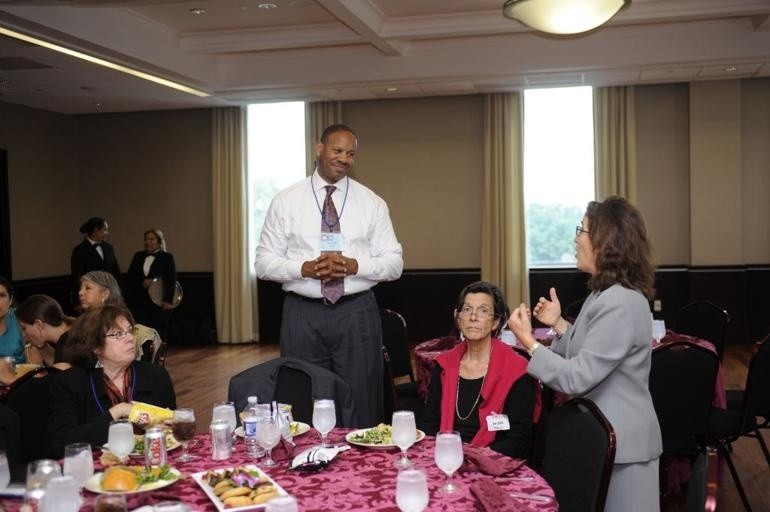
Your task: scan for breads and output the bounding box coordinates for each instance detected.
[216,477,277,507]
[101,467,137,491]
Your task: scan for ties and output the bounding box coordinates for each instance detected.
[320,185,343,306]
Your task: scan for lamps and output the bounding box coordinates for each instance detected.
[502,0,630,36]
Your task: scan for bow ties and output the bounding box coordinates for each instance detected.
[93,242,103,248]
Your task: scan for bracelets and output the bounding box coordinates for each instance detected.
[527,341,539,357]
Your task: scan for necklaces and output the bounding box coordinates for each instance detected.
[456,361,489,420]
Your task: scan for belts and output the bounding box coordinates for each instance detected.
[287,290,368,305]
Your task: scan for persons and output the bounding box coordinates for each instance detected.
[421,282,542,462]
[71,218,120,315]
[45,304,176,457]
[256,124,403,428]
[15,294,77,371]
[127,229,176,344]
[507,196,663,512]
[79,271,136,358]
[1,276,44,387]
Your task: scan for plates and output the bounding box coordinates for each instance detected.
[235,420,311,438]
[101,432,184,460]
[15,363,41,377]
[190,465,289,512]
[80,464,182,496]
[347,426,428,450]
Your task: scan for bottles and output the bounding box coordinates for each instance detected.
[242,394,267,460]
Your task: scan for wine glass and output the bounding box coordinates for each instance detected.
[170,408,205,462]
[433,429,469,493]
[391,409,421,470]
[650,320,668,344]
[212,400,240,452]
[311,398,340,449]
[107,420,138,464]
[255,414,279,466]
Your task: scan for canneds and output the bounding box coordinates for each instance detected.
[144,428,167,469]
[209,420,232,461]
[1,356,16,374]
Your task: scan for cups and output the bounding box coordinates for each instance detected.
[266,495,299,512]
[93,494,127,512]
[394,465,431,512]
[501,330,517,346]
[154,501,186,512]
[27,439,94,512]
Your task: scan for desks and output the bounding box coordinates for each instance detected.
[7,430,559,512]
[413,329,716,412]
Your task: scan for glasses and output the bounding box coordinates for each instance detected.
[458,306,504,321]
[102,326,139,341]
[574,225,589,238]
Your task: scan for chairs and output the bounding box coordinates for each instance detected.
[231,356,355,428]
[533,398,617,512]
[5,317,162,484]
[648,301,769,510]
[382,310,420,416]
